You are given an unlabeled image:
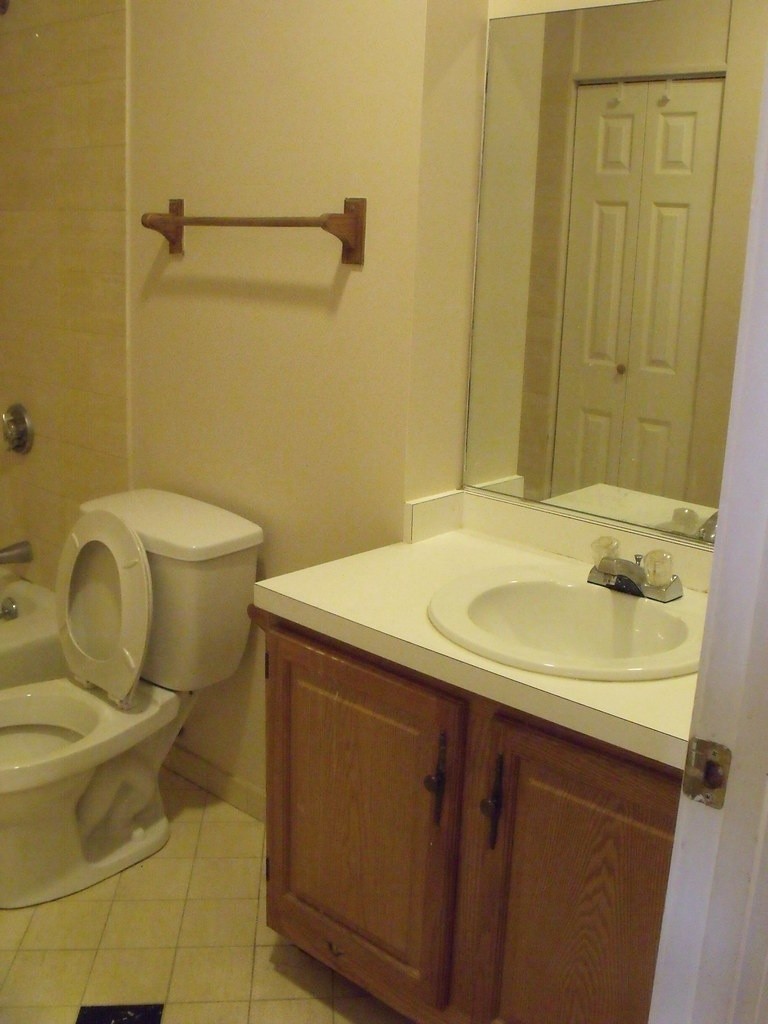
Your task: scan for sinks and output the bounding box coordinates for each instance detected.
[425,560,711,683]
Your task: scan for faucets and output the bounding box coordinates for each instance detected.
[587,553,683,603]
[692,509,721,545]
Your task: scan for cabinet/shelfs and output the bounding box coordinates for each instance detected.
[261,627,683,1023]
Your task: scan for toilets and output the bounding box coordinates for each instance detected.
[0,488,265,912]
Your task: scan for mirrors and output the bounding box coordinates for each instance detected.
[461,0,768,546]
